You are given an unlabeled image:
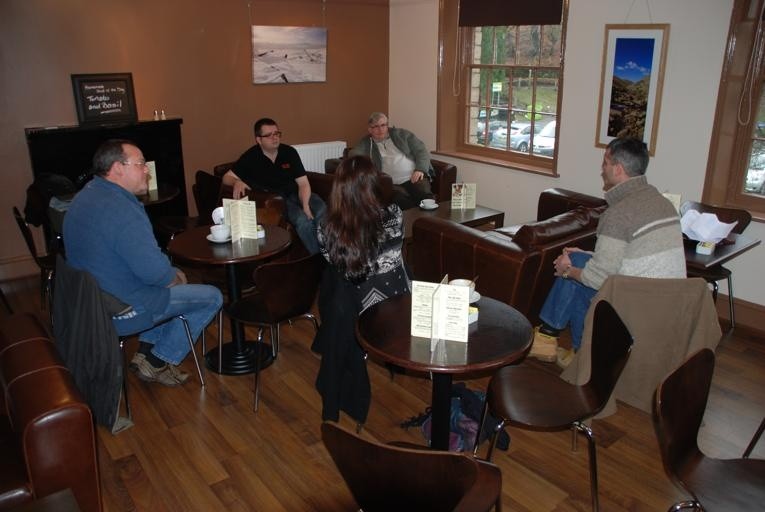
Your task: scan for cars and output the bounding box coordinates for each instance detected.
[753,120,765,144]
[478,106,556,156]
[741,146,765,196]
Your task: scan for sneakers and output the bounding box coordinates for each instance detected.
[135,356,194,389]
[522,324,560,364]
[554,345,580,373]
[128,352,152,370]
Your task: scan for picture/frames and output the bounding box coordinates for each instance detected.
[73,74,139,125]
[595,23,671,156]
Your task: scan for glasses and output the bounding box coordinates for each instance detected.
[258,131,282,139]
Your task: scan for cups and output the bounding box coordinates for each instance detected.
[448,279,475,299]
[420,199,435,207]
[210,224,231,240]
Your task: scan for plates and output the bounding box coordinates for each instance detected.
[469,291,481,304]
[206,234,232,243]
[419,204,439,210]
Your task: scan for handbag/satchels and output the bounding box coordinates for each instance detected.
[419,389,493,454]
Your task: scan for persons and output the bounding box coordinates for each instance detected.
[63,140,226,388]
[222,117,330,256]
[316,153,411,314]
[522,135,688,373]
[346,112,436,212]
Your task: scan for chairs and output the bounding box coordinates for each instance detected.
[218,252,321,412]
[12,206,203,420]
[470,300,633,511]
[679,200,752,328]
[652,348,763,512]
[320,422,503,511]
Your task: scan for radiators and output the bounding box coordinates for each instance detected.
[290,139,348,175]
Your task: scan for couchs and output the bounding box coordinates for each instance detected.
[192,162,333,239]
[411,187,609,328]
[325,147,457,211]
[1,291,105,510]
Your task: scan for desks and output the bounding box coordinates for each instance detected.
[683,232,762,269]
[166,222,293,376]
[354,291,533,451]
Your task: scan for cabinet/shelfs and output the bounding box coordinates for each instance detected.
[26,113,188,254]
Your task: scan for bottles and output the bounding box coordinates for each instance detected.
[153,109,166,121]
